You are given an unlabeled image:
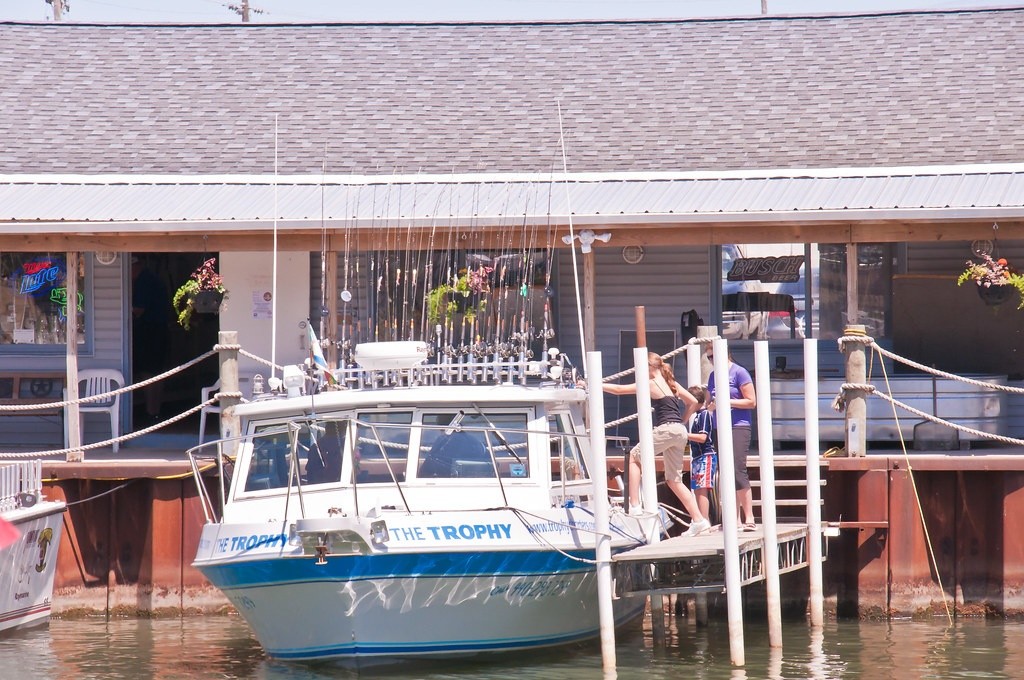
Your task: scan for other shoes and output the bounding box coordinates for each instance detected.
[743,523,757,531]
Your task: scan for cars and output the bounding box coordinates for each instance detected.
[765,268,820,340]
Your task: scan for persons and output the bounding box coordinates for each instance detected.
[304,421,348,483]
[419,415,501,476]
[576,352,711,537]
[707,339,760,531]
[0,302,18,343]
[688,386,719,535]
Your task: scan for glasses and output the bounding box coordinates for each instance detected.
[707,355,713,359]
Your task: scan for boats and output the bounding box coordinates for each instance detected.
[190,102,653,672]
[0,460,68,634]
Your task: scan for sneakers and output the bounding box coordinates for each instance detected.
[621,502,643,516]
[681,518,711,537]
